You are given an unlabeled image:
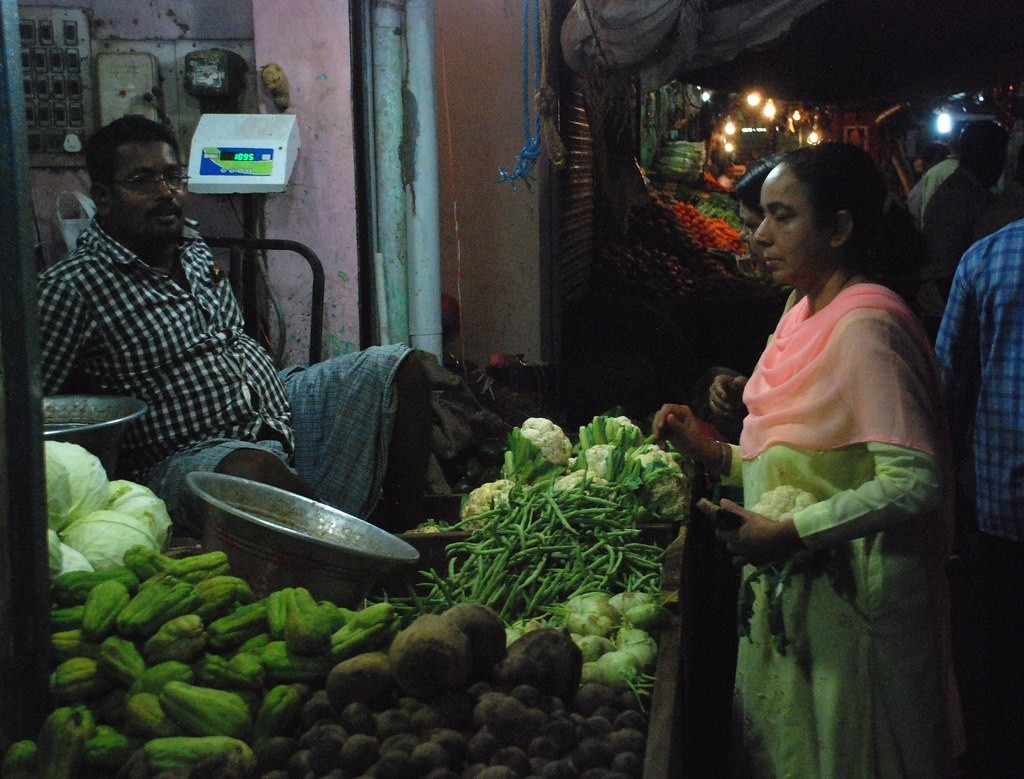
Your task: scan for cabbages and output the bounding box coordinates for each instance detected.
[45,440,172,580]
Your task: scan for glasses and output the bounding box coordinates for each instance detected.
[113,173,191,193]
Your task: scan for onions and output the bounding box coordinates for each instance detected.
[593,208,734,301]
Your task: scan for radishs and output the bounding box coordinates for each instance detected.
[500,591,671,690]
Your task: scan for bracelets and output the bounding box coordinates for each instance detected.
[690,437,725,477]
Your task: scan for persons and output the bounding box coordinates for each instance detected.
[922,119,1010,301]
[709,153,786,420]
[933,147,1024,779]
[969,144,1024,245]
[35,114,473,536]
[651,140,965,779]
[906,143,951,229]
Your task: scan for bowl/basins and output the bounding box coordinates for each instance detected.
[185,471,421,606]
[37,394,151,481]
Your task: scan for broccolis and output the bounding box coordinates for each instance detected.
[403,414,690,534]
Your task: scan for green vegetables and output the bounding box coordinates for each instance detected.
[654,139,709,176]
[696,193,742,226]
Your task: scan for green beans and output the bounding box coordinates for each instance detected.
[363,459,672,630]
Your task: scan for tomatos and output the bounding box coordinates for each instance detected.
[668,201,748,256]
